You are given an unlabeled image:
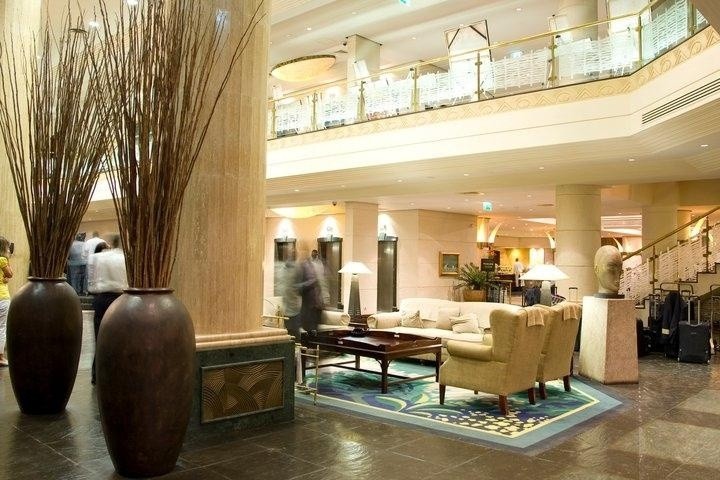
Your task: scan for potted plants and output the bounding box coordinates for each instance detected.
[453,262,499,301]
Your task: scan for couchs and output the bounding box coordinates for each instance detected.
[367,298,523,366]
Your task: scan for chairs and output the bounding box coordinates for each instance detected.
[439,301,582,415]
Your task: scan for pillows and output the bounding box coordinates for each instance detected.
[399,308,422,329]
[435,302,460,330]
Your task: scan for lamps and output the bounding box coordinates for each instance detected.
[613,237,631,256]
[477,213,503,249]
[269,54,337,83]
[517,265,570,306]
[338,261,373,315]
[269,202,328,219]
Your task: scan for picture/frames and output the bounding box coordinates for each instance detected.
[438,251,460,276]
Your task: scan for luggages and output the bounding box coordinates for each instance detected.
[678,296,711,364]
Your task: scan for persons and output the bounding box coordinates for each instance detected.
[66,238,86,296]
[108,232,124,256]
[514,257,525,289]
[86,226,111,254]
[86,242,131,386]
[0,236,15,368]
[280,239,327,345]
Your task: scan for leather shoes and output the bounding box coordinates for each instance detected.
[0,358,9,366]
